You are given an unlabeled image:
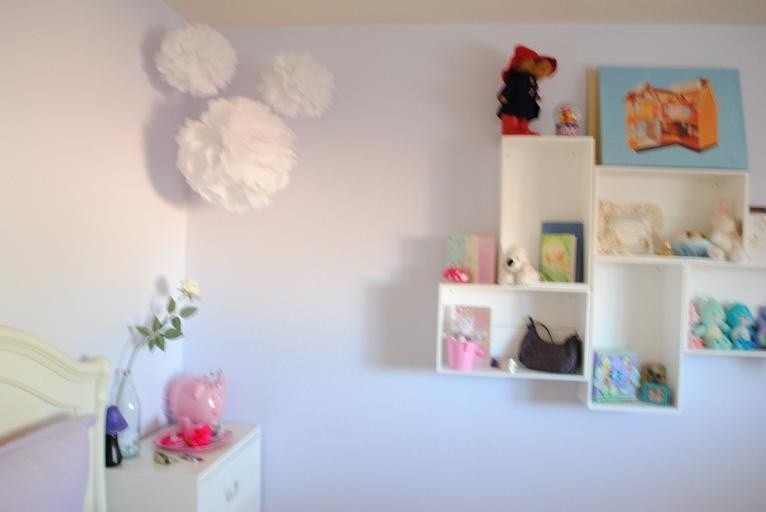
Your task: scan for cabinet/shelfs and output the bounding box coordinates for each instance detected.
[434,135,766,414]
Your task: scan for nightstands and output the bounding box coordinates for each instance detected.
[106,422,261,511]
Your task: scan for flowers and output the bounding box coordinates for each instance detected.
[117,278,202,405]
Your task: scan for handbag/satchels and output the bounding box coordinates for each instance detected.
[519,317,582,374]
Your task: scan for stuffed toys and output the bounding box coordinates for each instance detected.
[551,99,585,138]
[497,45,559,137]
[598,197,747,263]
[638,363,670,405]
[687,294,766,352]
[503,243,541,286]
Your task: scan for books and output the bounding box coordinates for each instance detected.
[539,231,578,283]
[538,221,586,284]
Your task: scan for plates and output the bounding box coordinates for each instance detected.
[154,427,234,453]
